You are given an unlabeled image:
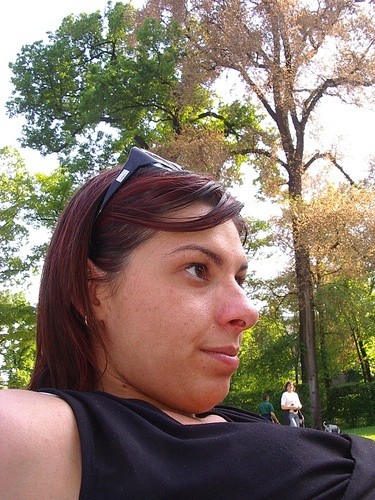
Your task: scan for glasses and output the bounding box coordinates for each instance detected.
[93,145,181,230]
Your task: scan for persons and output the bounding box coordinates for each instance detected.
[258,393,280,426]
[280,381,303,427]
[1,146,375,500]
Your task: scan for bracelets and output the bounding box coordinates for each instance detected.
[277,421,280,424]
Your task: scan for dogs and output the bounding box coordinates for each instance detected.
[322,421,342,434]
